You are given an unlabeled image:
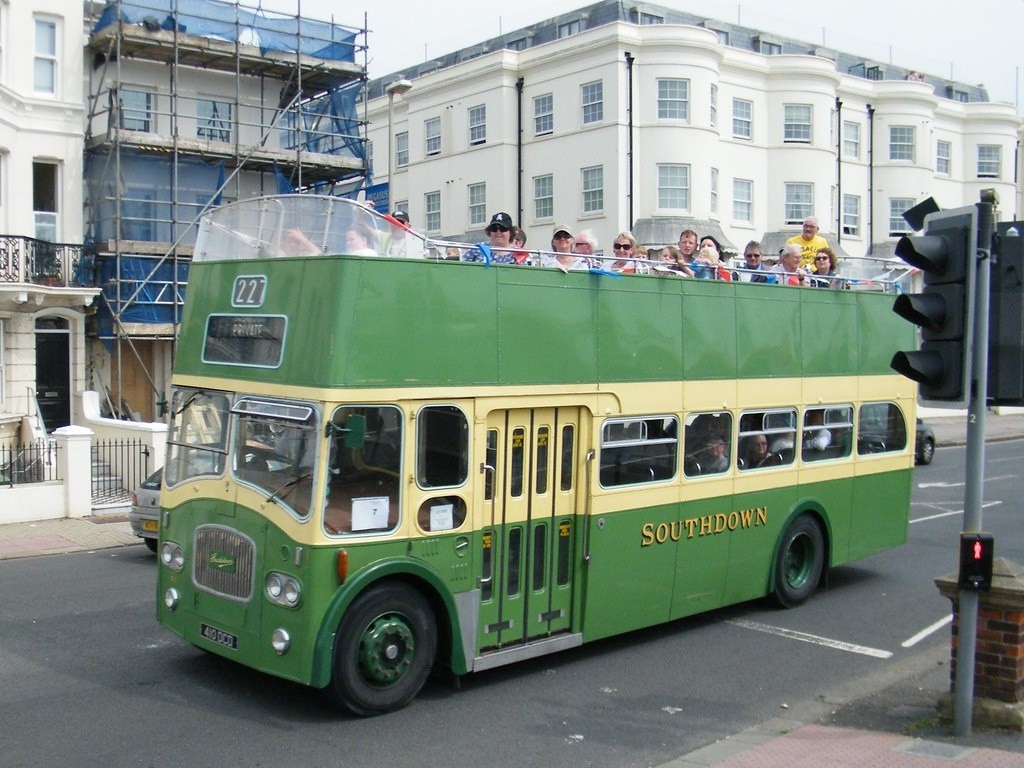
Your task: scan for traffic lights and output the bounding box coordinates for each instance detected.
[890,204,979,409]
[958,530,994,588]
[990,220,1023,409]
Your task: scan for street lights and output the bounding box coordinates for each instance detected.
[386,79,412,217]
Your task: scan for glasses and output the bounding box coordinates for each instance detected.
[746,253,760,258]
[575,242,588,247]
[490,225,508,233]
[803,224,817,228]
[614,243,631,250]
[816,256,828,261]
[754,441,768,447]
[396,219,405,225]
[553,231,570,240]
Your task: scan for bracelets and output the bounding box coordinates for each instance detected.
[799,278,804,281]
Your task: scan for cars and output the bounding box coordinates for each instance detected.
[864,411,935,464]
[130,446,283,556]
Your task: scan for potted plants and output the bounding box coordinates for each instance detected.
[32,239,62,286]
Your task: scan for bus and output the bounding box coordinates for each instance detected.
[156,194,926,718]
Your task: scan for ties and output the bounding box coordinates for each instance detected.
[293,420,310,468]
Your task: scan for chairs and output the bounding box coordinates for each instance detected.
[613,441,885,482]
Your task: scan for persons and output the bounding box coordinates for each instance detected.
[602,409,842,472]
[444,212,541,266]
[285,231,322,256]
[344,224,378,256]
[356,200,430,258]
[774,216,840,286]
[250,410,337,480]
[543,224,602,268]
[602,230,730,281]
[745,434,772,468]
[732,240,777,283]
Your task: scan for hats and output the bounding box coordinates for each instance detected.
[489,213,512,228]
[392,210,409,222]
[552,225,572,237]
[694,247,719,265]
[778,246,784,253]
